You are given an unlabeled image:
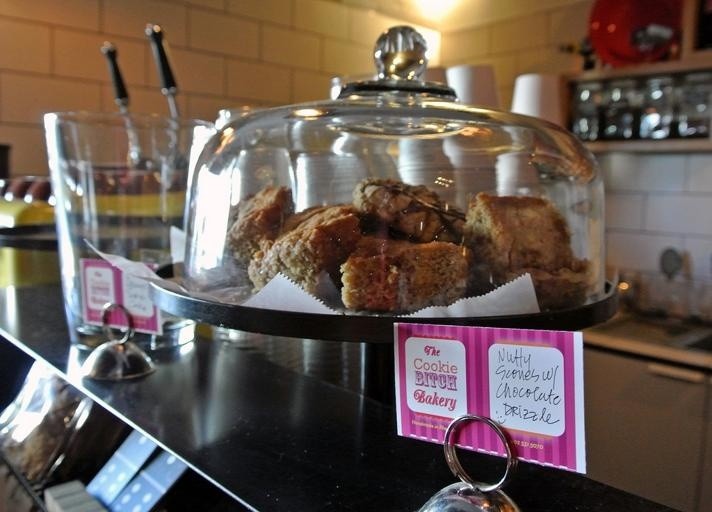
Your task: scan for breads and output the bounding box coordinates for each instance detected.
[226,185,296,268]
[464,194,589,301]
[342,233,469,310]
[352,177,467,242]
[248,204,359,295]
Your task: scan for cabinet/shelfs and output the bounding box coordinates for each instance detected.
[581,313,711,512]
[559,52,711,153]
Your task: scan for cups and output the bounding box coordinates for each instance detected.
[43,109,196,351]
